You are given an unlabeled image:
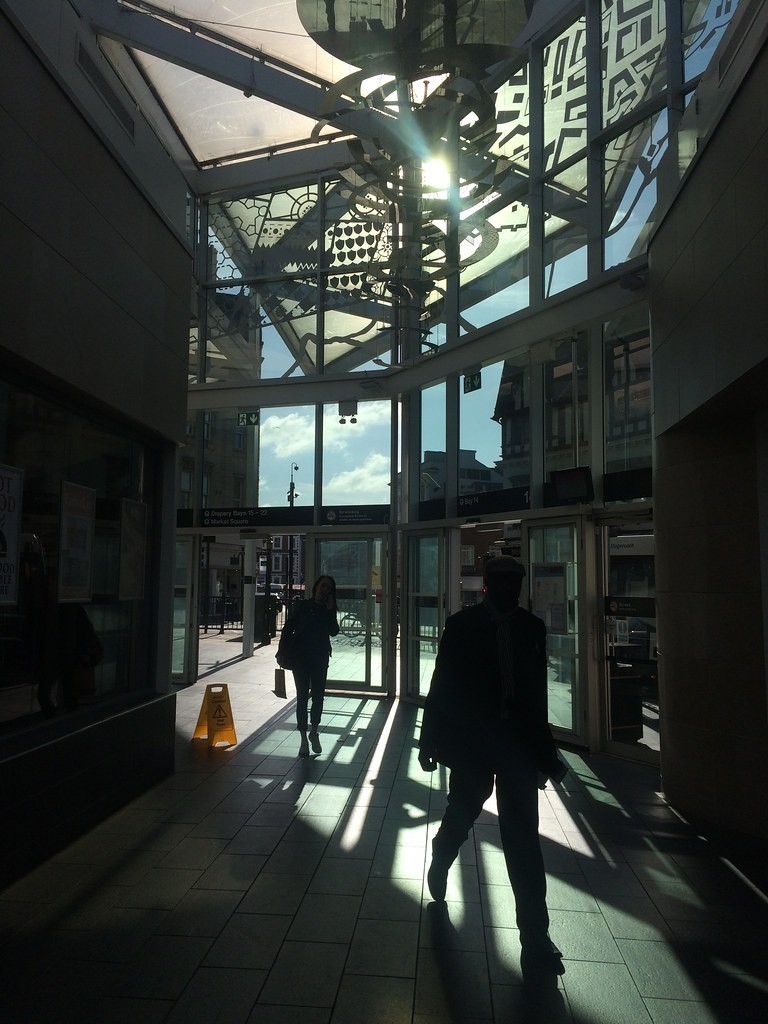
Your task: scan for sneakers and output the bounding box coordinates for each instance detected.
[308,730,322,753]
[299,738,309,756]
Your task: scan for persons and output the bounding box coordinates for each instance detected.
[275,575,340,757]
[418,555,563,959]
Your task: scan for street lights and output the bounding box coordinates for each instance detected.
[287,462,299,619]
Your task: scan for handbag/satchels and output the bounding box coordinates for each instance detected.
[272,666,287,699]
[279,633,305,666]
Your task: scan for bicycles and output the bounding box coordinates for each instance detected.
[340,600,399,641]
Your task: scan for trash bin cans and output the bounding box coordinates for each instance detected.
[254,593,277,643]
[609,641,645,743]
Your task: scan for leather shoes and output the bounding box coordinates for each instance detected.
[428,853,449,903]
[520,930,564,959]
